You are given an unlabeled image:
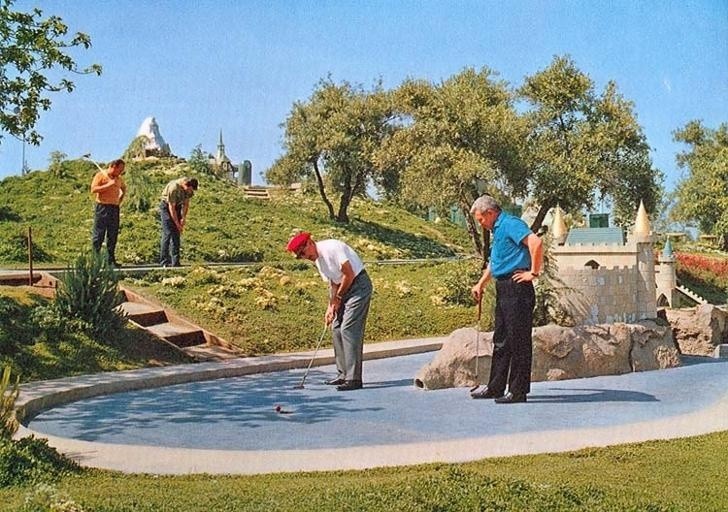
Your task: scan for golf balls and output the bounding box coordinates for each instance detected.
[275,405,280,412]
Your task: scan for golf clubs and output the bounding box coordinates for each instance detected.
[470,292,481,393]
[82,154,123,198]
[292,320,329,389]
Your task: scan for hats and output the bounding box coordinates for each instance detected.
[288,233,310,252]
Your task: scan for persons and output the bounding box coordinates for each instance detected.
[467,193,543,405]
[156,176,199,267]
[286,229,373,391]
[91,158,128,268]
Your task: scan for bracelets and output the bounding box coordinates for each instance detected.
[335,294,342,303]
[530,271,539,277]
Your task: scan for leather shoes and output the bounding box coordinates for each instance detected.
[336,379,362,391]
[326,378,345,385]
[470,386,504,399]
[495,392,527,403]
